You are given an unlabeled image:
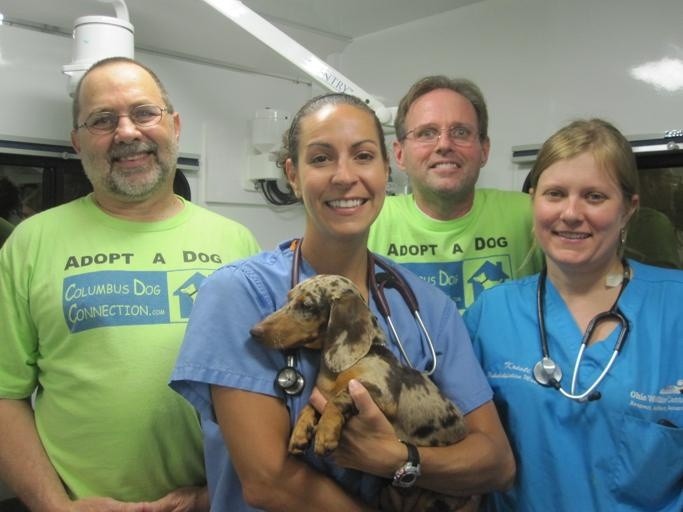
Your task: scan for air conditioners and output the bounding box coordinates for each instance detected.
[245,272,494,512]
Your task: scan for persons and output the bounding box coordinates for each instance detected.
[0,56,266,512]
[620,166,683,269]
[366,74,547,316]
[462,118,683,512]
[168,92,517,512]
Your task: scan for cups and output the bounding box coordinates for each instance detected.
[77,105,168,135]
[398,123,483,145]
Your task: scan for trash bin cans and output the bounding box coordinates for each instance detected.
[392,440,421,488]
